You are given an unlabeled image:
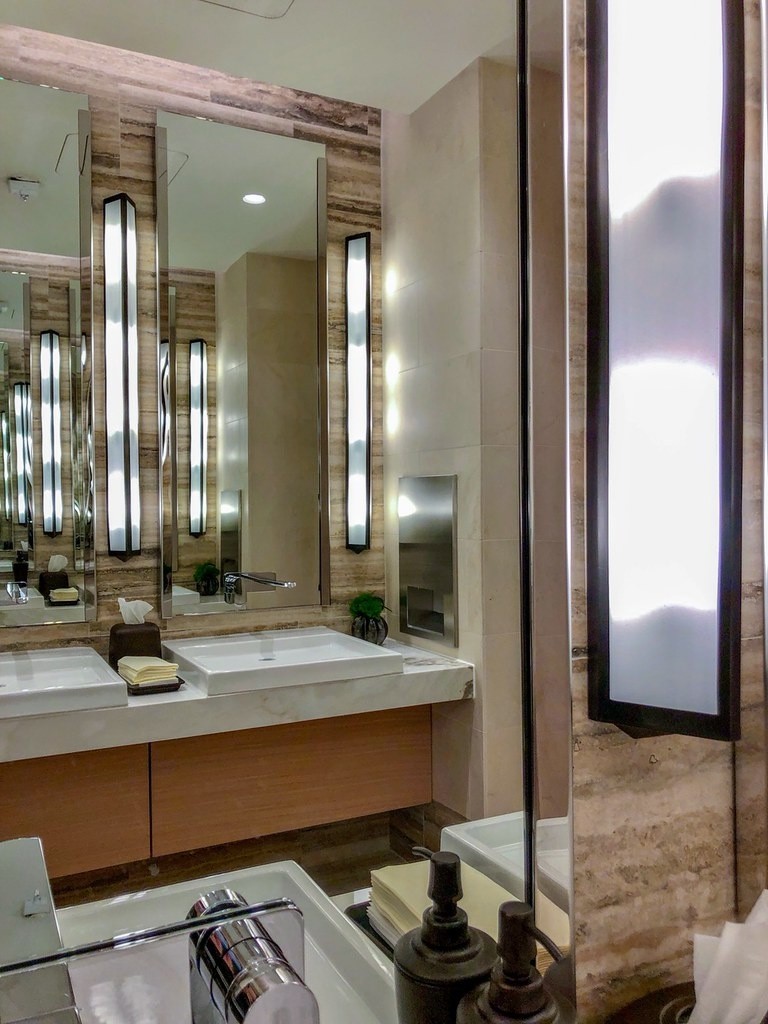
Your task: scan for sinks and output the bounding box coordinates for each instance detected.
[161,623,405,697]
[440,807,527,903]
[0,645,128,724]
[48,857,414,1024]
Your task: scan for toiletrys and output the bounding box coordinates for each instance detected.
[390,845,503,1024]
[10,548,29,585]
[450,900,578,1024]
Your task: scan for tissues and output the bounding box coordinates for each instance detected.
[37,554,73,598]
[109,598,163,673]
[15,540,31,560]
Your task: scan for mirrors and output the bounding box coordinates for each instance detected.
[395,474,459,647]
[150,107,335,614]
[1,75,100,628]
[3,0,583,1021]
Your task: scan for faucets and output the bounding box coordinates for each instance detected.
[224,570,298,611]
[6,578,29,606]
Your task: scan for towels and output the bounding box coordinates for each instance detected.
[115,655,180,687]
[362,854,575,987]
[48,587,80,604]
